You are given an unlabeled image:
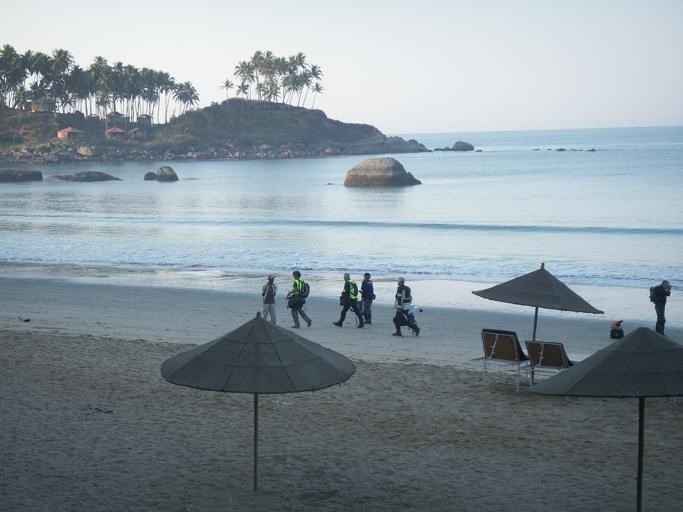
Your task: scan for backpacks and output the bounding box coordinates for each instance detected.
[298,279,309,297]
[650,286,658,303]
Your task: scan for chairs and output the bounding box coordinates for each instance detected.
[470,329,527,391]
[524,339,579,387]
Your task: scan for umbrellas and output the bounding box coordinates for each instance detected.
[473,263,604,342]
[161,312,355,492]
[528,326,682,511]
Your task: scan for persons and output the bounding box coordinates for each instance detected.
[288,270,311,327]
[261,273,276,325]
[392,278,420,337]
[650,280,671,334]
[333,273,362,328]
[357,273,373,324]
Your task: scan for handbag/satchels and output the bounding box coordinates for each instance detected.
[288,294,305,308]
[393,311,407,325]
